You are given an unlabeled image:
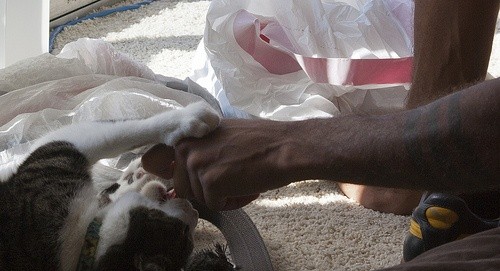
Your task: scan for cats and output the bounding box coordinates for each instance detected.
[0,100,222,271]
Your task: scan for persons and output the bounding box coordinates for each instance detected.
[141,0,500,271]
